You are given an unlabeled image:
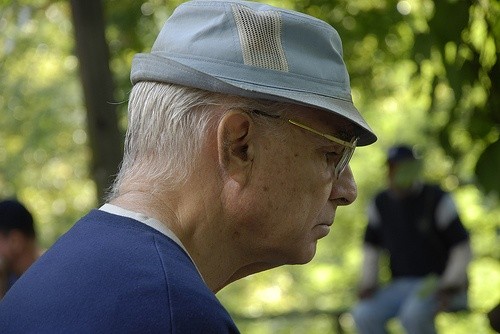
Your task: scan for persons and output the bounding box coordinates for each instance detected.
[0,197,44,301]
[352,143,472,334]
[0,0,377,334]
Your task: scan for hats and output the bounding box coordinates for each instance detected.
[129,0,377,146]
[383,145,416,163]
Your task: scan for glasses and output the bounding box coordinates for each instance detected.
[227,108,360,178]
